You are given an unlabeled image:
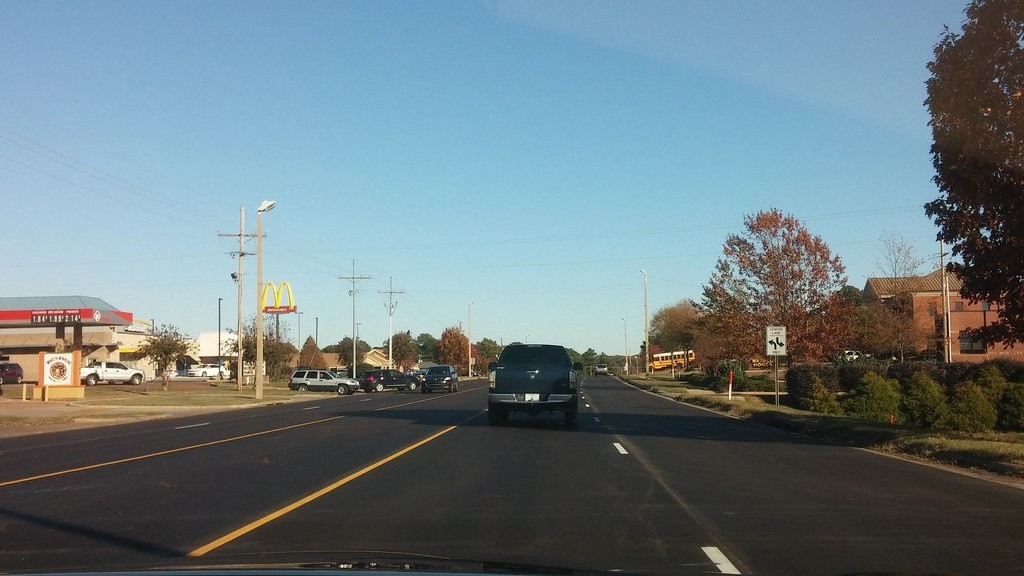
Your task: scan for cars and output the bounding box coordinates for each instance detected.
[187,364,224,378]
[404,368,429,383]
[218,365,235,378]
[161,369,181,377]
[594,364,608,376]
[358,369,421,393]
[837,351,871,363]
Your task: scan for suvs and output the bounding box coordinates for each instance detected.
[288,370,360,395]
[420,364,459,393]
[0,362,23,385]
[487,342,584,426]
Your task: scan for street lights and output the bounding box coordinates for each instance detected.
[469,300,474,379]
[255,200,278,399]
[621,318,628,374]
[640,269,649,373]
[218,298,223,373]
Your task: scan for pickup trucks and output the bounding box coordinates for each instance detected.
[80,361,145,387]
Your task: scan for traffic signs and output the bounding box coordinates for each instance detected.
[765,326,787,356]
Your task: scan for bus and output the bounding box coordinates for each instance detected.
[648,350,696,371]
